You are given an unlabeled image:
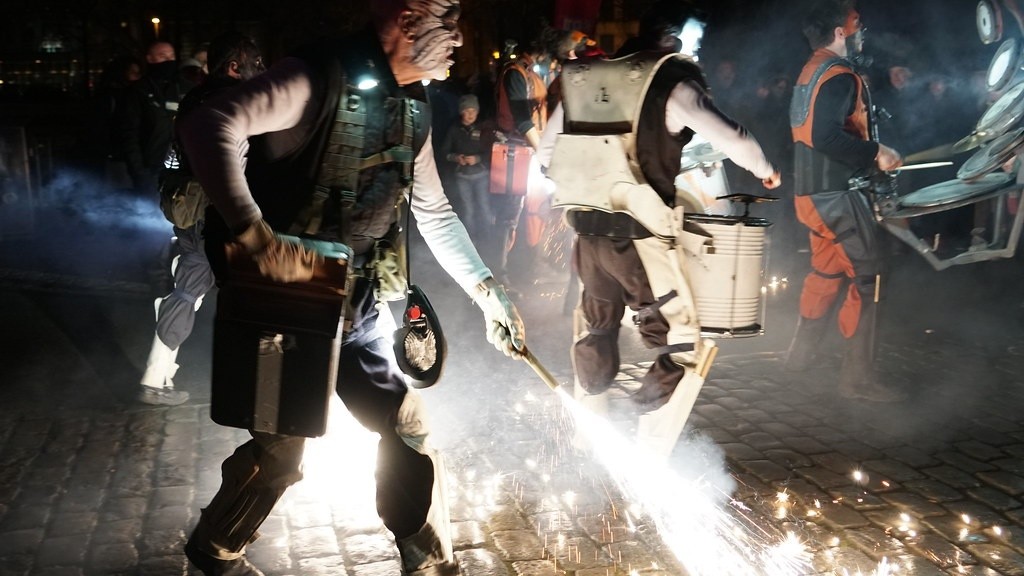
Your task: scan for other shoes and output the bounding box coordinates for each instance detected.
[183,544,266,576]
[783,351,841,372]
[839,372,910,402]
[400,553,466,576]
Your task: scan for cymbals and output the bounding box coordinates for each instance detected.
[950,126,1000,156]
[714,192,781,204]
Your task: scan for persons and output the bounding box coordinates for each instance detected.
[97,38,213,190]
[548,0,790,477]
[785,0,911,403]
[138,26,272,408]
[189,0,538,576]
[441,37,1008,316]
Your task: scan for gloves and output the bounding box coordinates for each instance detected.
[229,219,318,284]
[474,284,525,362]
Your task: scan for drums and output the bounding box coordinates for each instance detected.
[899,170,1019,275]
[974,1,1006,47]
[956,126,1024,184]
[985,37,1024,96]
[975,79,1023,134]
[679,211,776,340]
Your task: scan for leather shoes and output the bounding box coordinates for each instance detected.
[135,386,191,406]
